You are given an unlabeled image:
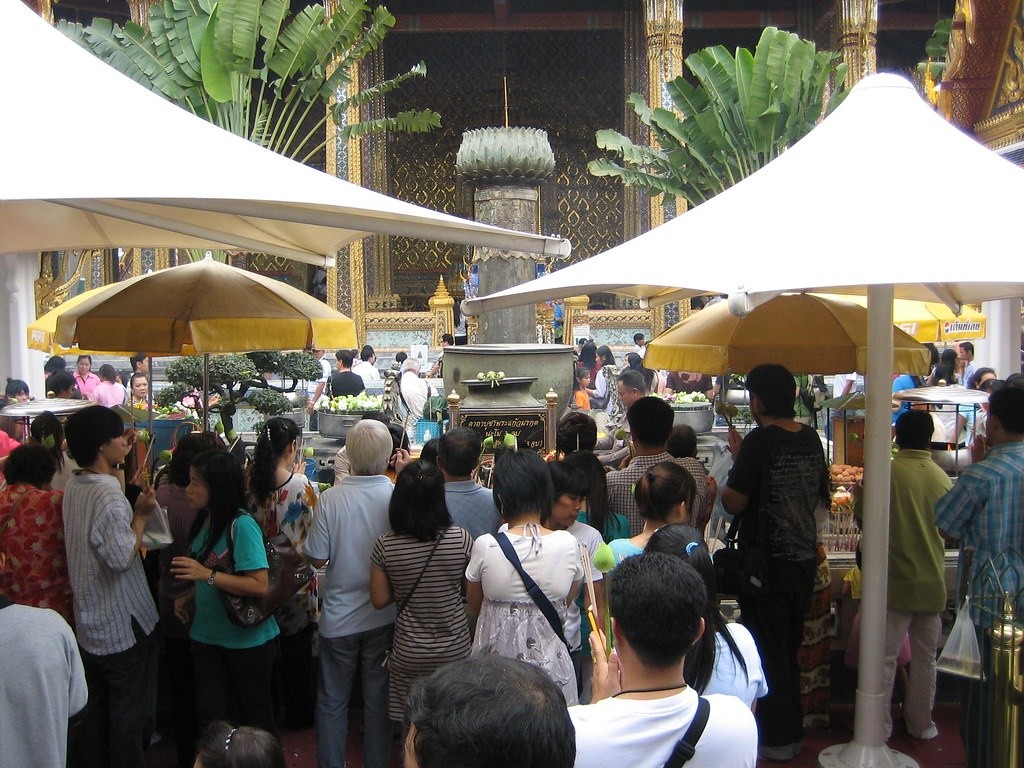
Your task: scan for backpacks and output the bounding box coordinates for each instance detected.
[801,373,828,411]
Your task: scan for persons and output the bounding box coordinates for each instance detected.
[400,654,576,768]
[465,447,590,706]
[170,448,289,768]
[369,460,474,722]
[853,410,953,739]
[301,418,396,768]
[0,299,1024,768]
[720,364,825,761]
[567,551,759,768]
[62,405,161,768]
[933,388,1024,768]
[194,720,287,768]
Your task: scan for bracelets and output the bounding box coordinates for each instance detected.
[112,463,126,469]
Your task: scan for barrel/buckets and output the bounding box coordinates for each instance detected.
[304,458,316,481]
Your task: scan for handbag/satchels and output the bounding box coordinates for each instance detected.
[589,398,609,409]
[712,548,743,595]
[217,511,308,627]
[933,596,984,681]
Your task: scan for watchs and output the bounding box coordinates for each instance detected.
[207,569,216,585]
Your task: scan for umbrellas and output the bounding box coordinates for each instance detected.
[53,250,360,431]
[780,291,986,343]
[642,292,930,377]
[458,73,1024,768]
[26,269,303,434]
[0,0,573,269]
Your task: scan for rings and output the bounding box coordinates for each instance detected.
[592,657,596,665]
